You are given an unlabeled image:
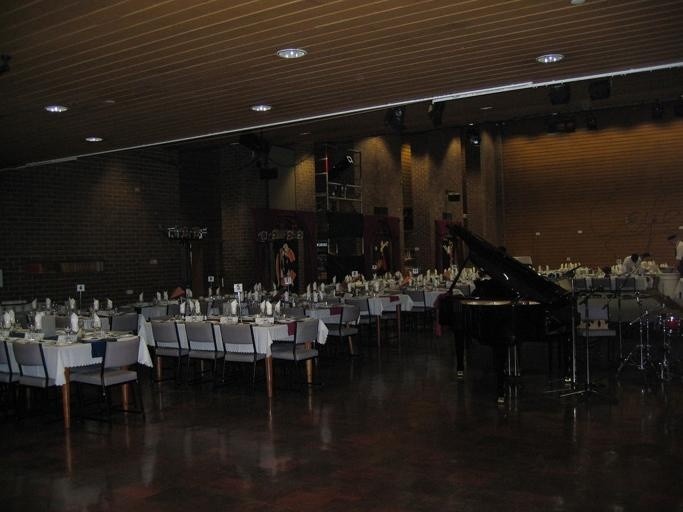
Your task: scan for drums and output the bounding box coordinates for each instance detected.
[654,313,681,335]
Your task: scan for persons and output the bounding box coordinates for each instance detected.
[667,235,683,278]
[642,252,662,274]
[623,253,644,276]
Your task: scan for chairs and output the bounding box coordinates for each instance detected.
[11,342,56,413]
[1,341,20,403]
[533,262,653,294]
[272,318,319,384]
[219,322,265,394]
[69,336,147,431]
[183,321,224,380]
[2,262,472,358]
[149,318,189,383]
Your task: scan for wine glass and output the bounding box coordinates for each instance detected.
[64,323,72,343]
[28,322,35,340]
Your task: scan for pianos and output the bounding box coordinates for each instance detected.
[433,224,581,404]
